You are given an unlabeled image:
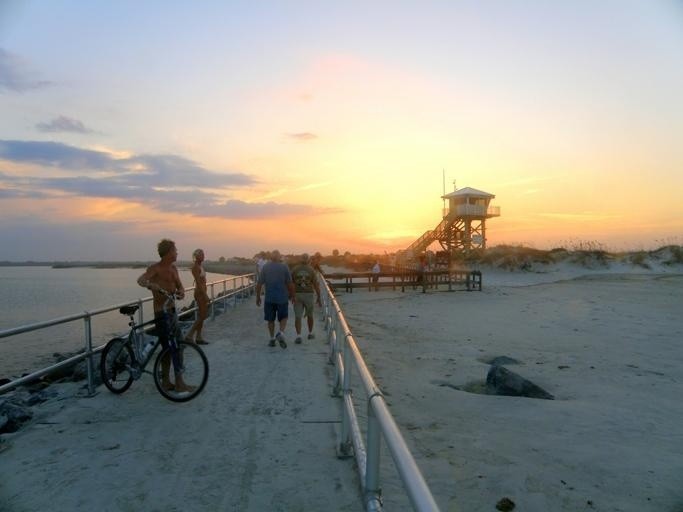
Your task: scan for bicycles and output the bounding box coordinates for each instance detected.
[97,284,212,404]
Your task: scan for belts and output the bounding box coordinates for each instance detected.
[295,290,314,294]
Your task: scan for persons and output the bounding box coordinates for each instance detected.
[183,247,211,345]
[253,250,295,348]
[287,253,322,344]
[370,260,381,292]
[253,248,325,296]
[414,256,426,294]
[135,238,199,394]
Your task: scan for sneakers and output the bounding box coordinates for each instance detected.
[268,339,276,347]
[196,339,209,344]
[185,334,195,343]
[276,332,287,349]
[308,334,315,339]
[295,337,302,343]
